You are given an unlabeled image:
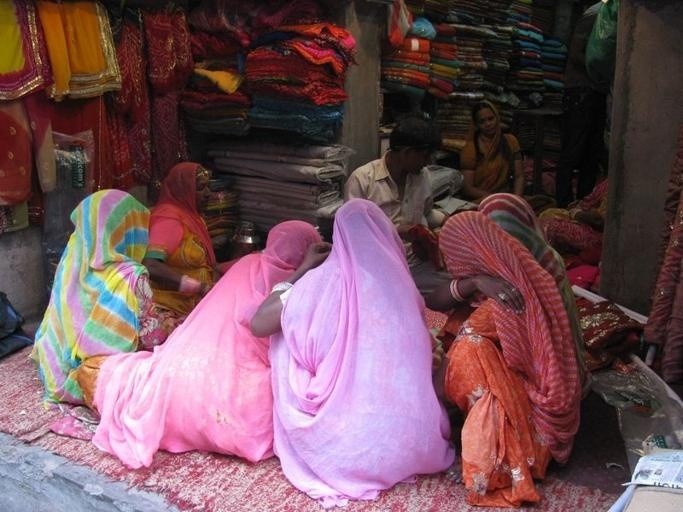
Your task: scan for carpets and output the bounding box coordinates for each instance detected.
[0,302,630,512]
